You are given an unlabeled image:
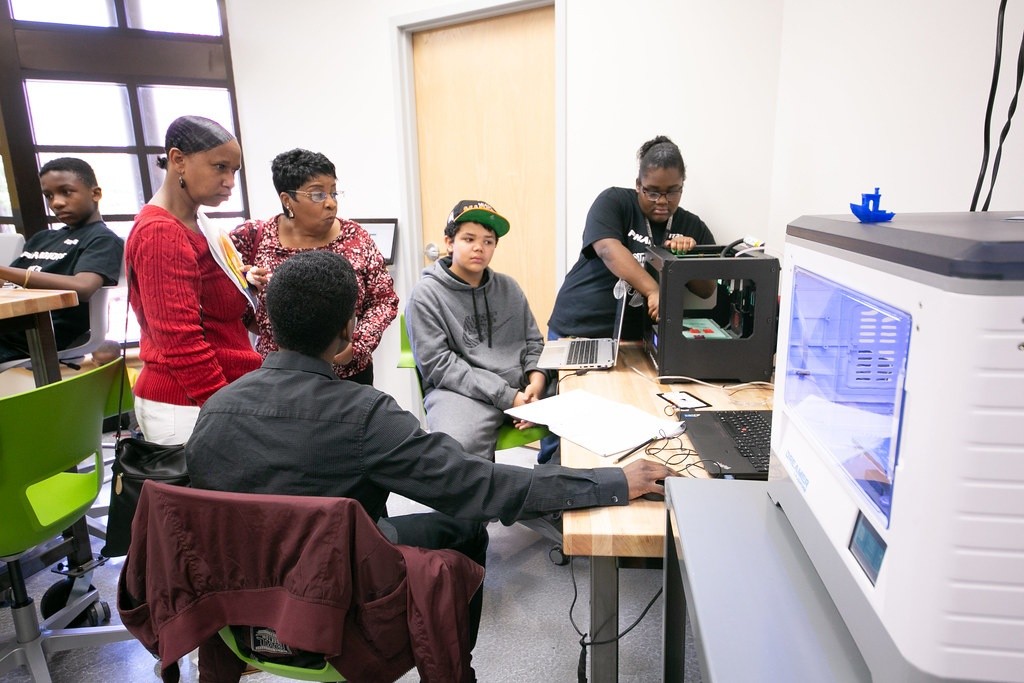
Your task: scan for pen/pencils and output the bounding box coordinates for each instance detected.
[614,435,659,463]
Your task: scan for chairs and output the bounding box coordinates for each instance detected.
[116,478,487,683]
[0,357,183,683]
[1,285,115,540]
[397,314,574,566]
[0,232,26,270]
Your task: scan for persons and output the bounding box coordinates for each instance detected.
[0,157,124,364]
[122,110,263,448]
[184,247,688,683]
[227,151,398,389]
[404,199,551,531]
[538,135,717,462]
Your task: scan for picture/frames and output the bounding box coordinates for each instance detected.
[348,219,398,265]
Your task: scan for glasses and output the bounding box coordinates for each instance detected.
[285,190,345,203]
[638,182,683,202]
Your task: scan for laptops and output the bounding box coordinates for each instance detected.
[676,409,773,480]
[535,283,627,372]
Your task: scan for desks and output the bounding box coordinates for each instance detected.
[558,339,776,683]
[0,287,110,629]
[663,476,874,683]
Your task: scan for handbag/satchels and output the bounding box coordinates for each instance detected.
[100,437,191,557]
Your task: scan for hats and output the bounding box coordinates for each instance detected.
[446,201,510,238]
